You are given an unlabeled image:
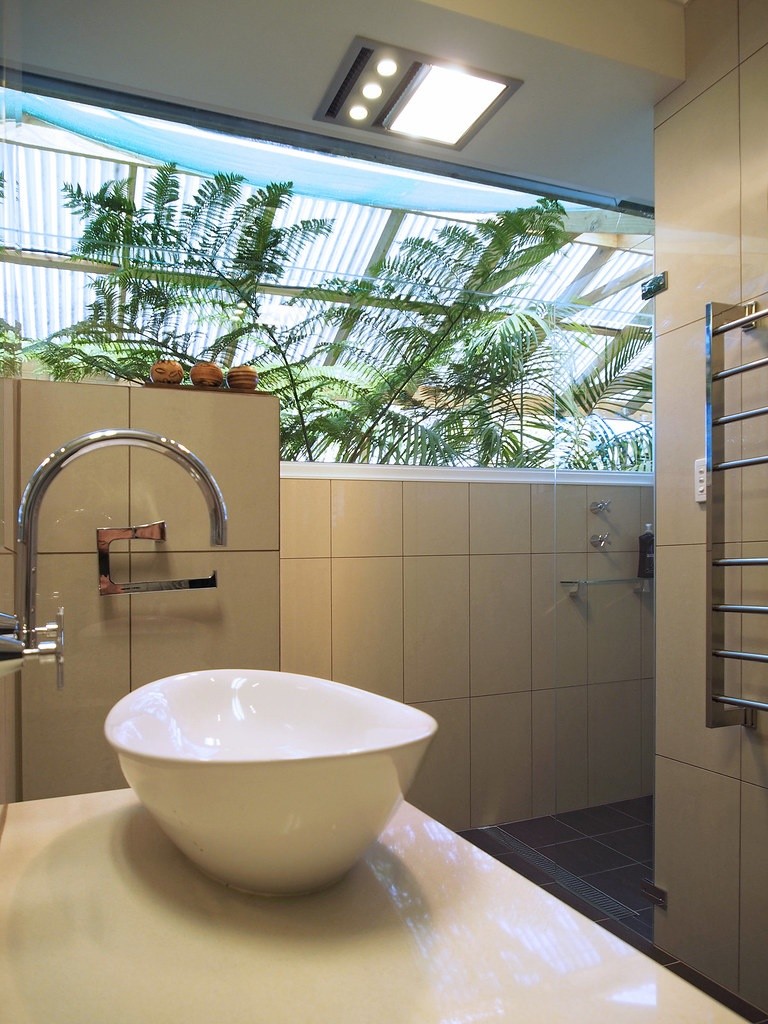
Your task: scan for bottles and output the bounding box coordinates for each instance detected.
[638,524,654,578]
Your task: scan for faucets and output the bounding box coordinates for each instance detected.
[13,427,228,650]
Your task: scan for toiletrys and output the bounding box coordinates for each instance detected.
[637,522,655,579]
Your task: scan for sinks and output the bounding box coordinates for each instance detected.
[102,668,440,899]
